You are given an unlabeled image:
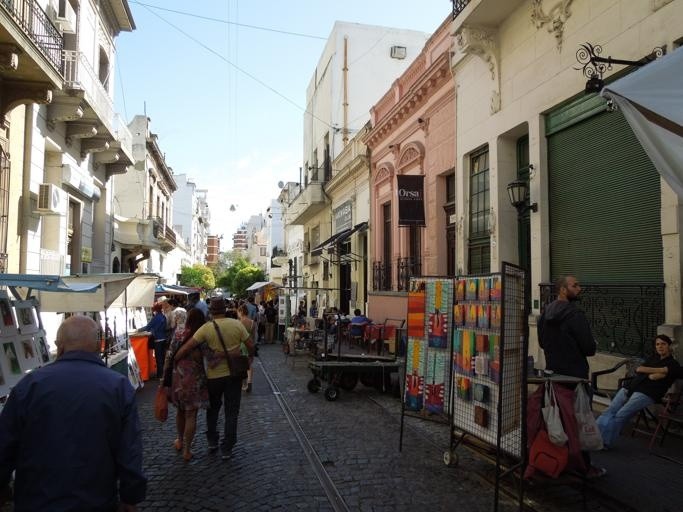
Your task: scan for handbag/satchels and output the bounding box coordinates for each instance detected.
[574,410,604,452]
[541,406,570,447]
[226,353,251,380]
[528,430,570,480]
[153,384,169,422]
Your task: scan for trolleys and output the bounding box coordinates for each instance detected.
[304,305,401,397]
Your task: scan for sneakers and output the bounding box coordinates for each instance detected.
[585,467,606,478]
[175,439,182,450]
[183,450,193,460]
[222,446,233,459]
[208,440,218,450]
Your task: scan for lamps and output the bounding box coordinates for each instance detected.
[506,176,537,213]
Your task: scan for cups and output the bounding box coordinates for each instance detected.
[537,370,544,378]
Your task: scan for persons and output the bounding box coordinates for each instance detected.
[319,304,350,334]
[596,334,680,452]
[310,300,318,318]
[133,294,282,391]
[351,309,373,336]
[294,311,309,338]
[173,297,254,460]
[298,299,308,319]
[537,275,596,467]
[159,308,211,460]
[186,292,209,317]
[0,314,148,512]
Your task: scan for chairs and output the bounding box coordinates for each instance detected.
[590,358,645,412]
[349,324,364,349]
[632,365,683,447]
[382,318,405,357]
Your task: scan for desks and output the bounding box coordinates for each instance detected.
[363,325,386,355]
[527,367,591,475]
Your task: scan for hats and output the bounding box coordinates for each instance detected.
[154,295,172,306]
[207,297,228,310]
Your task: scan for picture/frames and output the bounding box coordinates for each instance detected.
[0,290,18,337]
[14,301,40,335]
[15,335,40,370]
[0,337,26,386]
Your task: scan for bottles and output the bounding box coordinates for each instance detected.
[527,356,535,377]
[409,277,415,291]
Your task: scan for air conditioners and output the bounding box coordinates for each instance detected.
[37,183,67,217]
[56,1,77,35]
